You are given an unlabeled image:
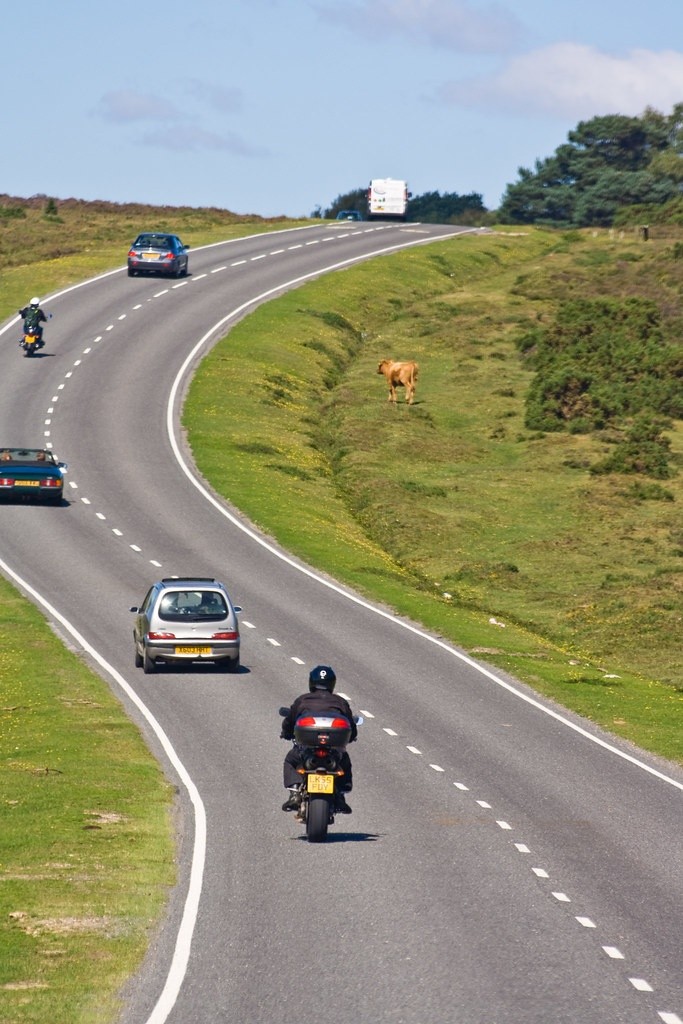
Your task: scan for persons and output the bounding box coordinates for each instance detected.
[18,297,49,345]
[1,453,11,461]
[280,666,358,813]
[36,451,45,463]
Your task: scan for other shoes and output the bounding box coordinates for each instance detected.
[334,796,352,814]
[282,794,301,812]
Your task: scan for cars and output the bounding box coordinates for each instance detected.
[0,447,68,505]
[127,232,189,279]
[336,210,363,222]
[129,575,243,674]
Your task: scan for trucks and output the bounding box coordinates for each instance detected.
[367,179,409,222]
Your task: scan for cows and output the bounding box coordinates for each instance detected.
[376,359,420,406]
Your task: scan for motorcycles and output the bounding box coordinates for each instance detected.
[19,310,53,358]
[280,705,364,843]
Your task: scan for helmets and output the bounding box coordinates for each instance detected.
[309,666,336,694]
[30,297,39,308]
